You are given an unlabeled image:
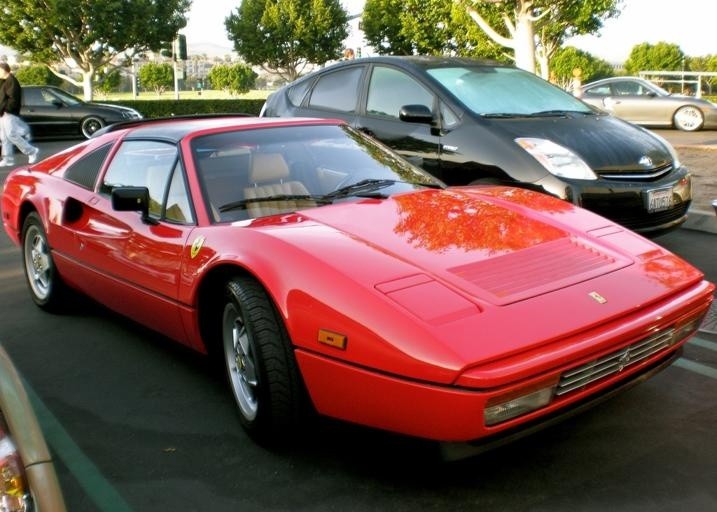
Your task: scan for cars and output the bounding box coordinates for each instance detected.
[19,86,143,139]
[260,59,695,241]
[564,76,717,132]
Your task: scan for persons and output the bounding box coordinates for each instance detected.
[0,63,39,167]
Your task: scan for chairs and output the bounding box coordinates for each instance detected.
[241,152,322,219]
[143,161,222,224]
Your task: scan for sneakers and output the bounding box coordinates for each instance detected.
[0,160,15,166]
[28,148,39,163]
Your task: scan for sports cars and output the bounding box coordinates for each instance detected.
[0,118,716,451]
